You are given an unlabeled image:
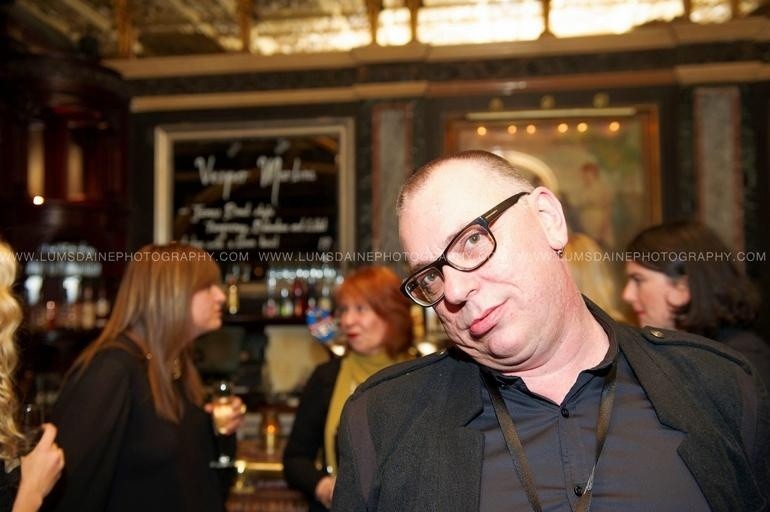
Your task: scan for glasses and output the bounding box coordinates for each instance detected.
[399,192,530,308]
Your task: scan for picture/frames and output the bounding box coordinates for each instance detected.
[152,116,356,297]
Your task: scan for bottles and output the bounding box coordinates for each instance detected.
[258,406,282,453]
[265,278,336,319]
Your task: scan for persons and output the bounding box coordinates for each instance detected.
[33,241,248,512]
[334,151,769,512]
[0,237,68,512]
[280,261,423,512]
[621,219,770,394]
[561,233,627,324]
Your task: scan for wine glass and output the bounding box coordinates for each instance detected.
[15,404,43,456]
[208,382,239,470]
